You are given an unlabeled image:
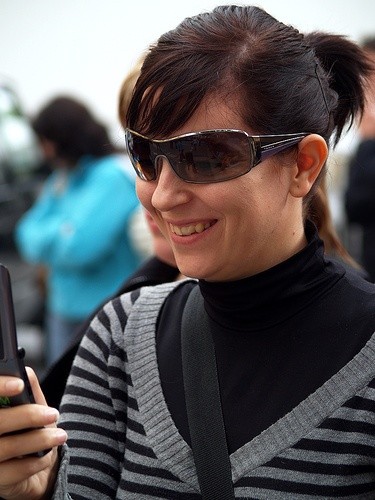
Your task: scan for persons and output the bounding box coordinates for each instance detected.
[1,5,375,500]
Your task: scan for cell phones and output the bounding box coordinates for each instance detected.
[0,264,52,459]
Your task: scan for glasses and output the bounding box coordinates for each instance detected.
[123,125,310,185]
